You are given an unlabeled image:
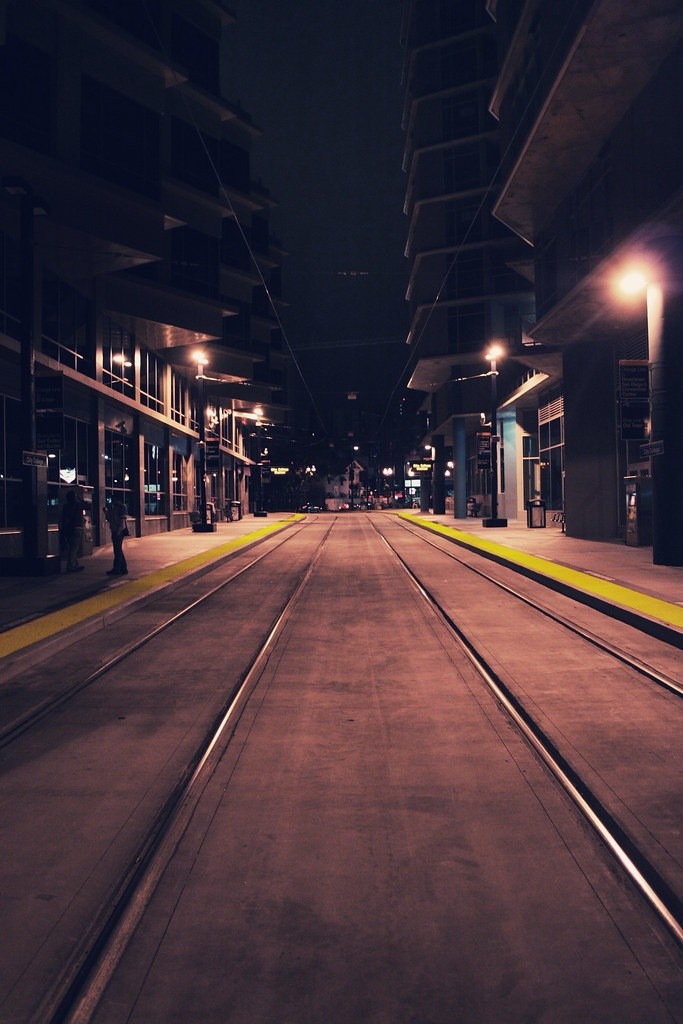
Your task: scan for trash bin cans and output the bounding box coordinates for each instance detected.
[525,500,547,530]
[231,501,240,520]
[205,500,216,524]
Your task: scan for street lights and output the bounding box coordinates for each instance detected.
[254,423,267,517]
[192,348,217,532]
[482,343,507,526]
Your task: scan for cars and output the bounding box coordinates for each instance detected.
[301,502,321,513]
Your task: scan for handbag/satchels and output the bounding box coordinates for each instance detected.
[122,519,131,536]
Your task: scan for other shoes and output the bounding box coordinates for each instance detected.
[110,569,129,574]
[72,566,85,572]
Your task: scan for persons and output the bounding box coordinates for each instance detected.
[63,490,92,572]
[102,494,128,577]
